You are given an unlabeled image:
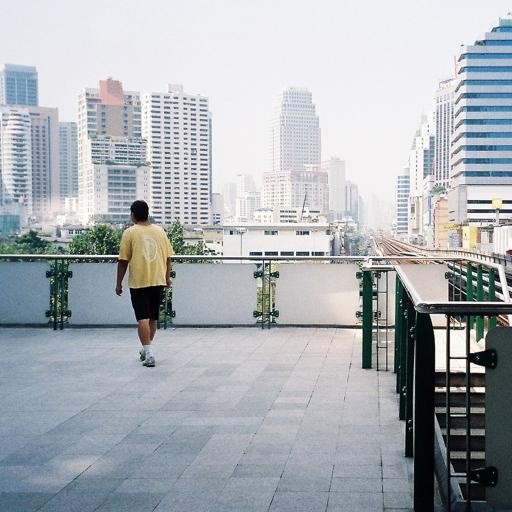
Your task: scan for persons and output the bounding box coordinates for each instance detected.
[115,201,175,367]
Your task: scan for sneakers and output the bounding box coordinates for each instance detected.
[140,350,147,361]
[143,357,155,367]
